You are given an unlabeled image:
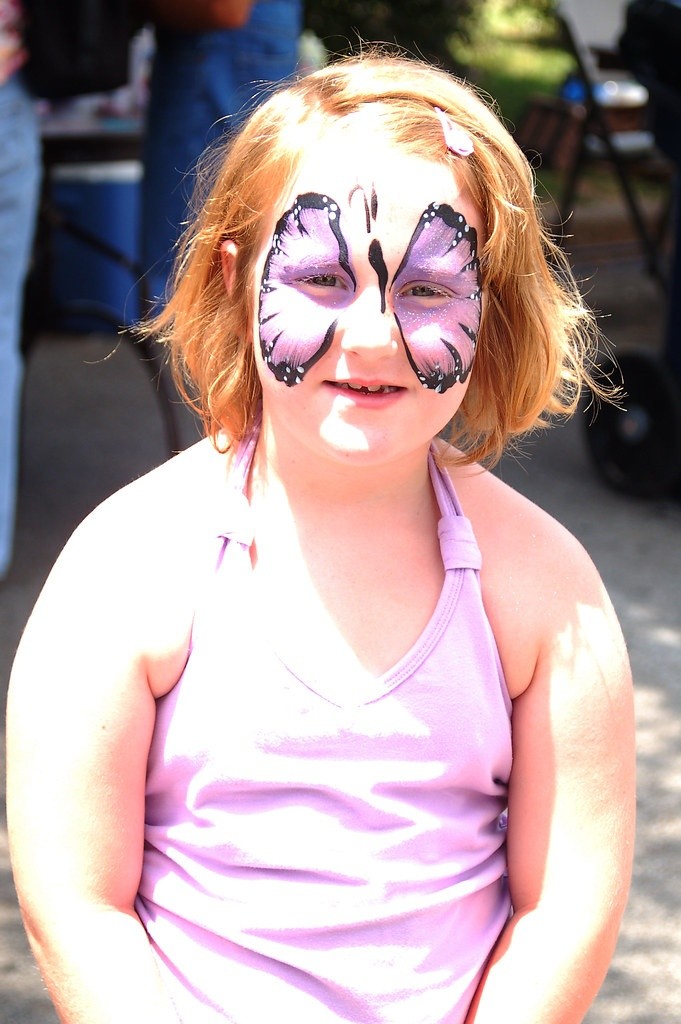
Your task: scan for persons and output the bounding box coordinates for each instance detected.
[138,0,301,357]
[0,0,41,599]
[6,39,636,1024]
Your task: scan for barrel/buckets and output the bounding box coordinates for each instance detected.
[50,160,144,337]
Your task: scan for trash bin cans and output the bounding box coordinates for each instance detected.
[46,161,143,337]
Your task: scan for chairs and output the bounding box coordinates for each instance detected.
[547,0,680,298]
[21,166,179,454]
